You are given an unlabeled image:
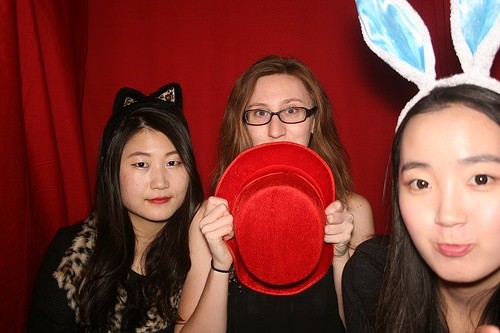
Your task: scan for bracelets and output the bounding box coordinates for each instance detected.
[211,258,232,273]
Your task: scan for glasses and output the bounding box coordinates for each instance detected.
[242,105,318,126]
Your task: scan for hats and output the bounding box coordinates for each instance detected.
[213,142,336,295]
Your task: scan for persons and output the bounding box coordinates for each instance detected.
[341,0,500,333]
[27,80,213,333]
[173,54,375,333]
